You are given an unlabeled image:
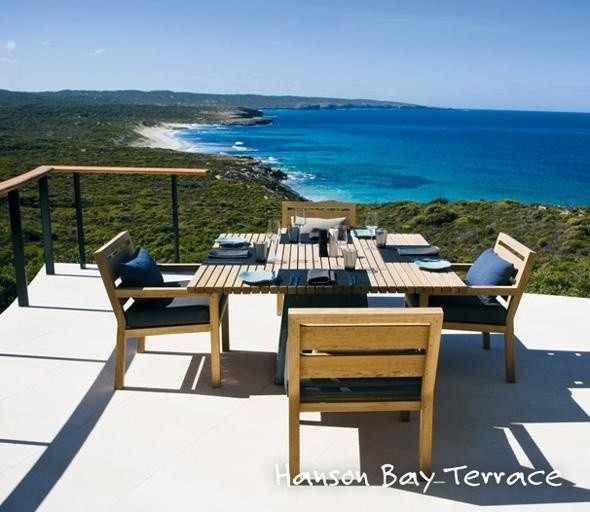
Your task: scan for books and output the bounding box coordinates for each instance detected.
[397,244,440,256]
[208,247,250,259]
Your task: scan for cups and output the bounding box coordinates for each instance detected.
[375,228,388,248]
[341,246,357,272]
[252,240,266,262]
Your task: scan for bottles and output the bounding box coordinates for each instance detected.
[318,228,340,259]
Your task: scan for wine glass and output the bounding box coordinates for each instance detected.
[365,212,378,246]
[266,218,281,264]
[292,207,306,246]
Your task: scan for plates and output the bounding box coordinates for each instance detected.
[238,269,279,284]
[214,238,248,248]
[354,229,380,237]
[414,259,451,270]
